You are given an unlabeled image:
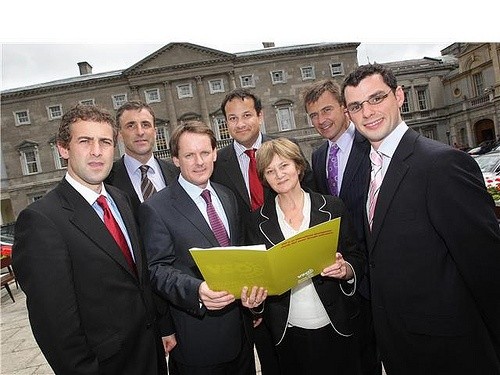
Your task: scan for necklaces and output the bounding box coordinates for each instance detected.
[278,198,305,224]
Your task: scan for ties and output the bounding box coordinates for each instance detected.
[244,149,263,210]
[368,152,382,231]
[141,167,157,202]
[97,196,135,274]
[200,189,230,248]
[328,144,340,198]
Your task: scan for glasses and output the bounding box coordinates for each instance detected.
[344,88,392,114]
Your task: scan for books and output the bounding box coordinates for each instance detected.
[189,217,341,299]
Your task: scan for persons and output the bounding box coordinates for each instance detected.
[209,88,318,375]
[302,79,372,374]
[451,135,500,151]
[340,61,500,375]
[102,101,181,375]
[12,105,179,375]
[137,120,263,375]
[241,137,371,375]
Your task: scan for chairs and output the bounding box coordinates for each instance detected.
[0,255,18,303]
